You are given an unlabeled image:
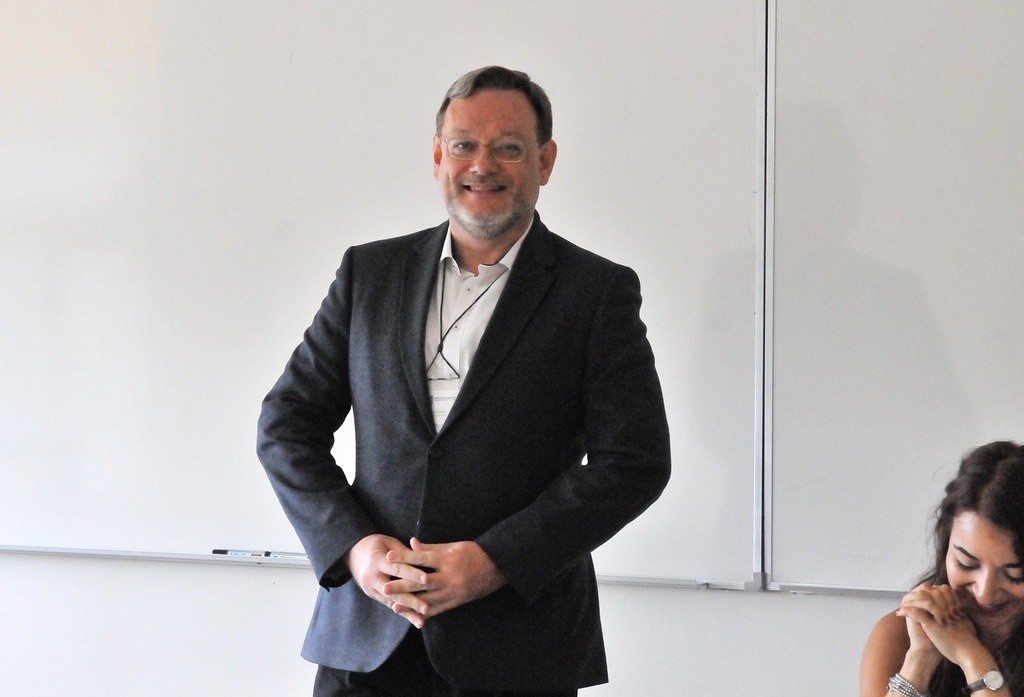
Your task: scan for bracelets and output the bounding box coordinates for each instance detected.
[886,673,930,697]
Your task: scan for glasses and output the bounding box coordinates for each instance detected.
[437,134,540,164]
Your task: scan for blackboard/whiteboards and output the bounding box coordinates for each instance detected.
[764,1,1024,598]
[2,0,765,595]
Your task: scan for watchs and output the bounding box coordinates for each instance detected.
[963,670,1004,693]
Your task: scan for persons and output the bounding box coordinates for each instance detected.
[860,441,1024,697]
[256,66,671,697]
